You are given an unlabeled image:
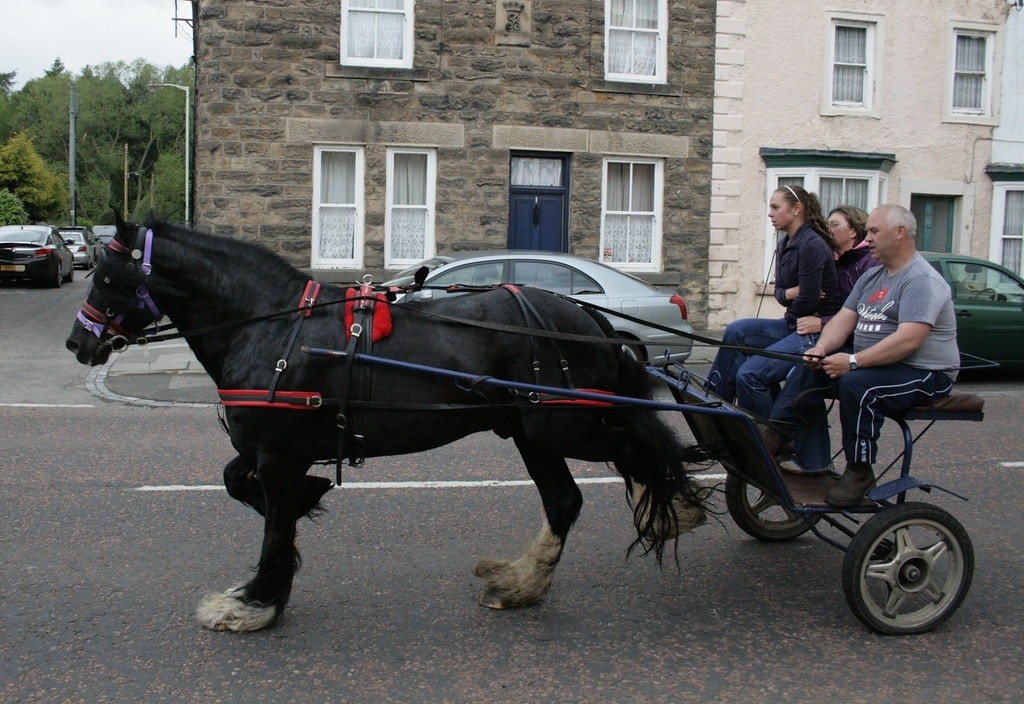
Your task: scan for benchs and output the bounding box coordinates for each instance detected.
[884,351,999,421]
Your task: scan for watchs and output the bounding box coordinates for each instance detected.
[849,355,858,370]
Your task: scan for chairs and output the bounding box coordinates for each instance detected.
[475,265,498,285]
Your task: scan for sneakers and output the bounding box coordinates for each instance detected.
[779,457,833,475]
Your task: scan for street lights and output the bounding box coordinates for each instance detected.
[146,83,189,228]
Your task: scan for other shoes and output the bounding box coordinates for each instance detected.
[824,463,876,507]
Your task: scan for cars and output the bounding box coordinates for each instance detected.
[376,250,695,367]
[0,224,118,288]
[916,250,1024,380]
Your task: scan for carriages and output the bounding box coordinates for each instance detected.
[66,202,1000,636]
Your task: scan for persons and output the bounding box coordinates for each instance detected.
[705,185,841,411]
[769,205,878,474]
[758,205,960,504]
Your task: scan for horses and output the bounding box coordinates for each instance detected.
[65,202,737,635]
